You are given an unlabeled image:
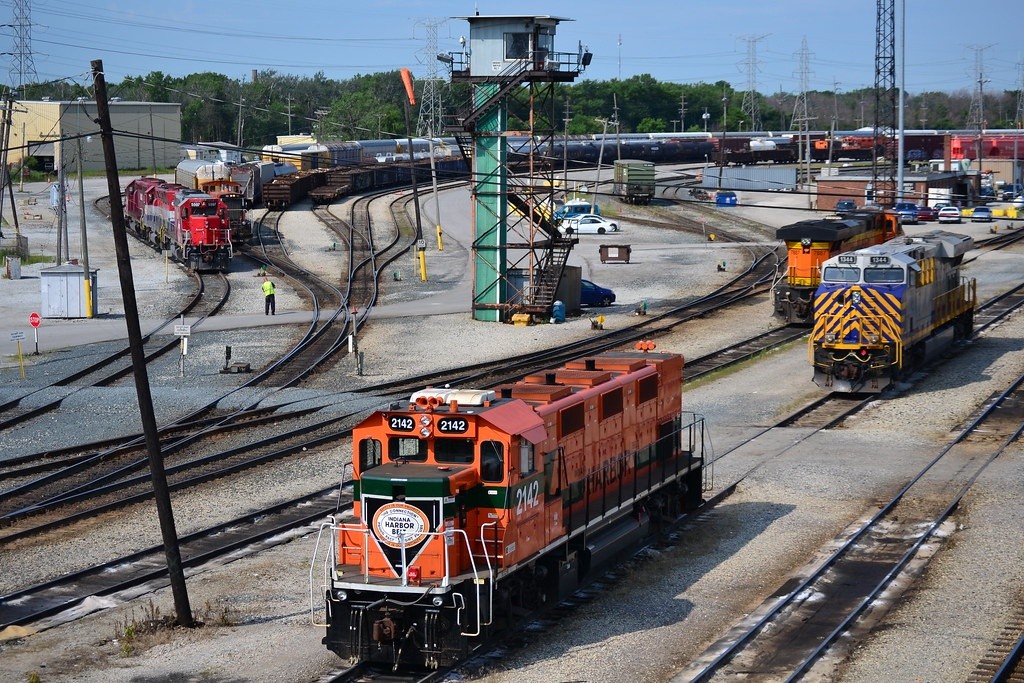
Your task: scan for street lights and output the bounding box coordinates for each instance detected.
[978,79,992,170]
[77,137,93,318]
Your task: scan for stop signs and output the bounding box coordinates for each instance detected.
[29,313,42,328]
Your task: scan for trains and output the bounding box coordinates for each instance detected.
[242,117,1024,210]
[176,160,255,246]
[771,209,905,327]
[310,337,716,675]
[806,229,978,393]
[123,177,233,272]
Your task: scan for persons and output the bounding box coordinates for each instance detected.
[261,278,276,315]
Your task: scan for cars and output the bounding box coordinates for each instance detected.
[971,206,992,223]
[557,217,613,234]
[981,187,994,201]
[564,214,621,232]
[917,205,935,220]
[933,202,949,216]
[938,207,960,223]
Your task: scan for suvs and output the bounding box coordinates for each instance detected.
[1003,184,1023,201]
[893,203,918,224]
[835,199,856,215]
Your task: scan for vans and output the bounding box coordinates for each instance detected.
[553,203,600,224]
